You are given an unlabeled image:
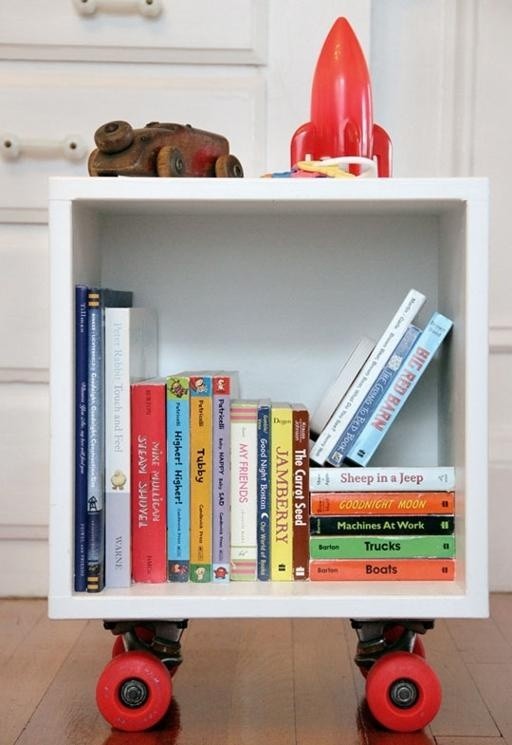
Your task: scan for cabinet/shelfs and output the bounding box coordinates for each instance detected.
[2,0,274,603]
[44,176,493,620]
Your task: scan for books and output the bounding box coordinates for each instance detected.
[75,284,455,593]
